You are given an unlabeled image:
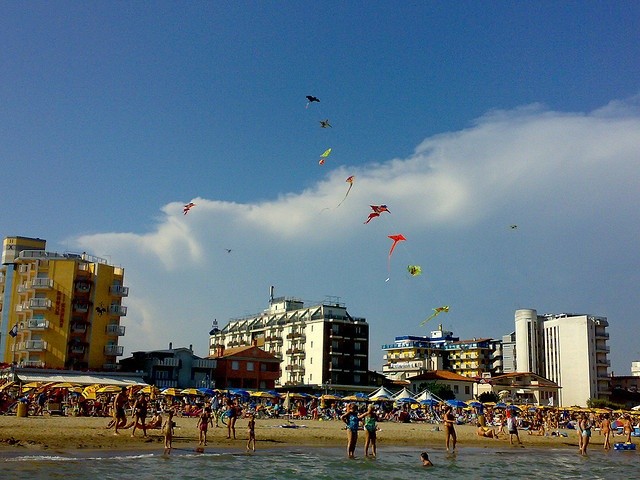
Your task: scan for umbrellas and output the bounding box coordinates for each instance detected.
[321,393,462,406]
[463,399,640,416]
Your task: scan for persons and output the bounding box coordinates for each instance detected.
[148,399,210,416]
[599,415,613,449]
[508,413,522,444]
[238,398,320,419]
[112,387,128,434]
[358,404,377,459]
[378,403,443,423]
[477,424,498,439]
[210,391,221,427]
[161,412,174,449]
[197,407,214,446]
[247,415,256,451]
[320,401,340,420]
[225,399,237,440]
[341,403,358,458]
[131,393,148,439]
[120,420,162,429]
[625,416,635,444]
[420,452,433,466]
[0,390,112,415]
[522,408,576,437]
[581,415,592,455]
[457,408,507,424]
[576,413,585,450]
[150,410,162,424]
[444,406,456,451]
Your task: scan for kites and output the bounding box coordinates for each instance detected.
[363,205,391,224]
[419,305,450,327]
[407,265,422,276]
[182,203,195,216]
[387,233,407,272]
[319,159,325,166]
[306,96,320,109]
[320,148,331,158]
[337,176,355,208]
[319,119,332,128]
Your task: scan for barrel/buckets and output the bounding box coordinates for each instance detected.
[17,403,27,417]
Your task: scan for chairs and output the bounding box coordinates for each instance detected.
[180,408,204,417]
[285,412,299,419]
[47,402,62,416]
